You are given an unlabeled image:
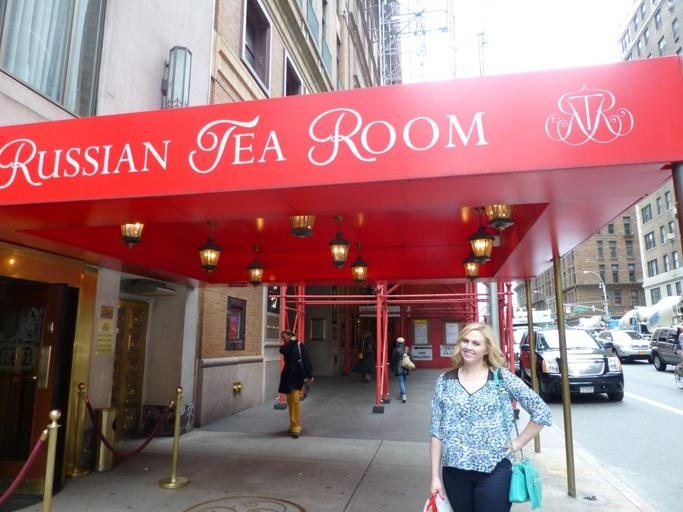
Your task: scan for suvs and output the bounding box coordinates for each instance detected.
[522,328,625,404]
[651,325,682,371]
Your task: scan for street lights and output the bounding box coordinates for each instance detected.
[583,270,609,319]
[533,290,550,310]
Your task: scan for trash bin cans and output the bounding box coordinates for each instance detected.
[91,406,118,472]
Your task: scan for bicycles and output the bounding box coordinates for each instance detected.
[674,351,683,389]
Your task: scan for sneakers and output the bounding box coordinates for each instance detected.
[401,395,406,403]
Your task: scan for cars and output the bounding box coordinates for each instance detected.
[508,326,542,374]
[596,329,650,363]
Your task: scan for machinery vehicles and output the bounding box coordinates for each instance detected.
[619,304,646,332]
[645,295,683,340]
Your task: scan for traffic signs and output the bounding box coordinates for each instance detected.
[565,306,570,314]
[573,306,590,311]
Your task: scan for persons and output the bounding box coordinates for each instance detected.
[278,329,318,439]
[391,336,416,403]
[355,330,376,384]
[429,323,553,512]
[675,324,683,377]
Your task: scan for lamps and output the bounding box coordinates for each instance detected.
[351,241,368,286]
[467,207,495,264]
[484,205,514,235]
[328,215,350,269]
[246,257,264,287]
[289,215,316,238]
[463,243,481,281]
[196,221,222,273]
[120,224,144,250]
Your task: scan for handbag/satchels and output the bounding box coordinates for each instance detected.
[402,354,416,370]
[508,459,542,510]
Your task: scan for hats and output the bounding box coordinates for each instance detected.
[397,337,405,343]
